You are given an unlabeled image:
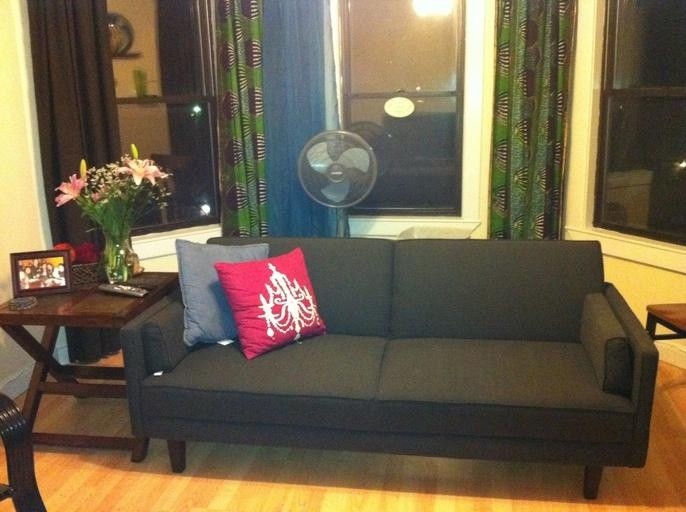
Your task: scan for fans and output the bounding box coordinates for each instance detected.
[293,128,380,238]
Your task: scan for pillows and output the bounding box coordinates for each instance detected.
[169,239,331,360]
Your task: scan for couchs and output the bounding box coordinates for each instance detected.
[120,236,660,503]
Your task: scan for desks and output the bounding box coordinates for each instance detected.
[1,263,178,465]
[645,297,685,361]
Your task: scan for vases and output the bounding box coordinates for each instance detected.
[101,233,131,286]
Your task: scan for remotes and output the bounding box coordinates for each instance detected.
[97,282,150,297]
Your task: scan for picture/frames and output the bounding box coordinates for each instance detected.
[7,249,74,299]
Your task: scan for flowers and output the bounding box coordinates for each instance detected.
[50,142,179,275]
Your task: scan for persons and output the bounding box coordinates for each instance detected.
[19,259,66,289]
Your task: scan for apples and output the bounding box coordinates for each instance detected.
[52,243,77,264]
[76,242,101,263]
